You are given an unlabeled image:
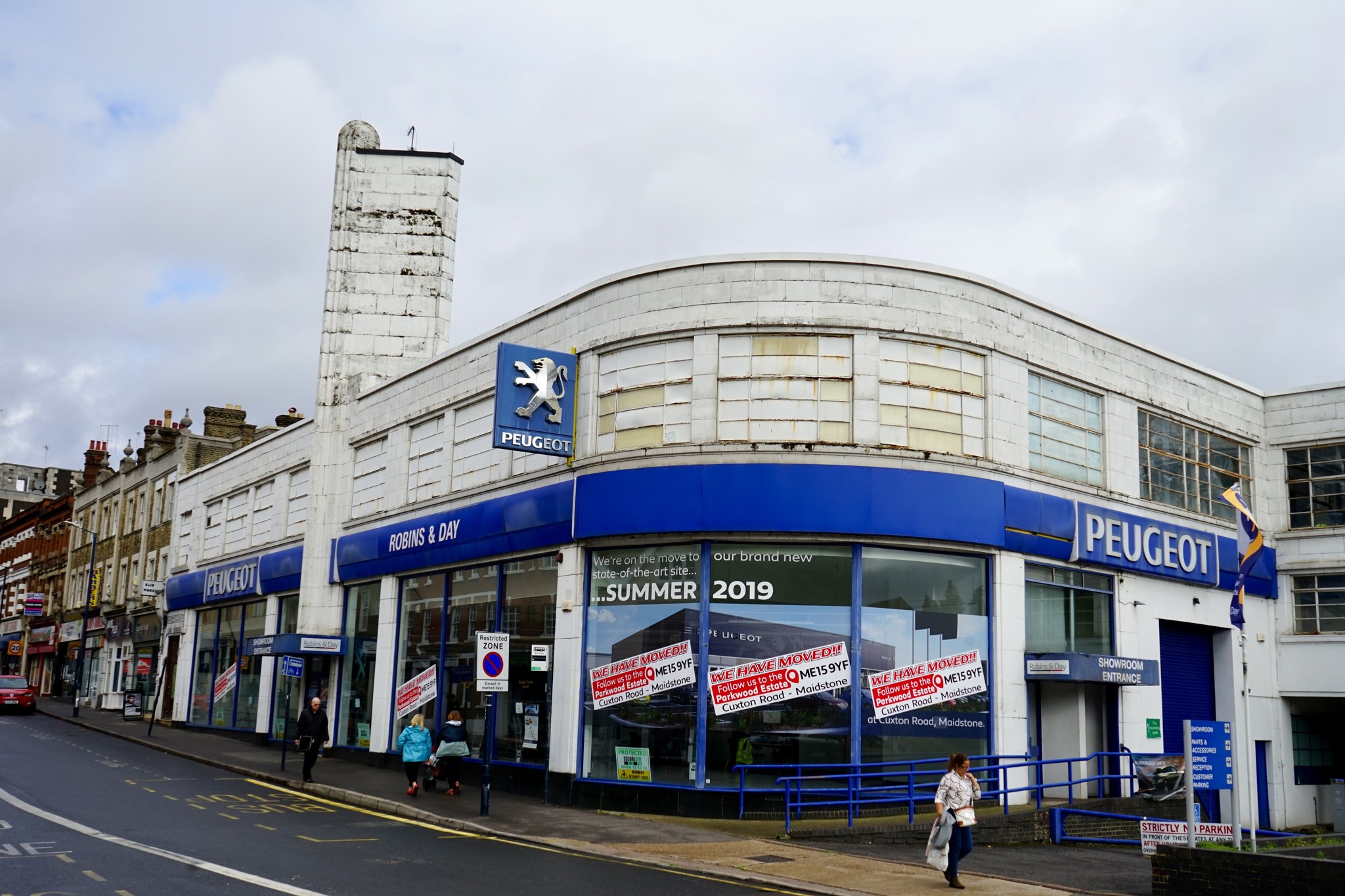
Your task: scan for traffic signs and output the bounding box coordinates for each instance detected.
[1191,720,1233,789]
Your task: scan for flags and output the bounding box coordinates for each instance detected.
[1220,482,1265,630]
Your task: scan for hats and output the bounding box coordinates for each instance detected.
[127,694,135,699]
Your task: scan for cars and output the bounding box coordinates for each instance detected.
[0,675,36,716]
[1152,765,1184,792]
[784,691,848,717]
[840,687,874,711]
[665,684,711,705]
[627,695,651,707]
[946,694,990,707]
[192,686,210,719]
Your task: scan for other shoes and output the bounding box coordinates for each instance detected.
[942,869,951,881]
[446,790,454,795]
[304,778,313,783]
[407,790,412,795]
[949,878,965,889]
[412,784,419,797]
[455,781,461,792]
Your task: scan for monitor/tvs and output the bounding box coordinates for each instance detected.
[525,704,539,716]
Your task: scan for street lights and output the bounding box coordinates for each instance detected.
[63,520,98,718]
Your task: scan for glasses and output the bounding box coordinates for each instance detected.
[314,704,320,706]
[958,763,971,770]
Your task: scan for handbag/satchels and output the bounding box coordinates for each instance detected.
[954,806,977,827]
[925,816,950,872]
[297,736,312,751]
[424,751,439,767]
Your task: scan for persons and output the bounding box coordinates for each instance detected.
[434,711,470,795]
[125,694,140,708]
[294,697,330,782]
[449,694,460,713]
[397,714,432,797]
[935,752,982,889]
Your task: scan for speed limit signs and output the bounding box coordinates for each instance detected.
[67,640,80,660]
[7,641,23,656]
